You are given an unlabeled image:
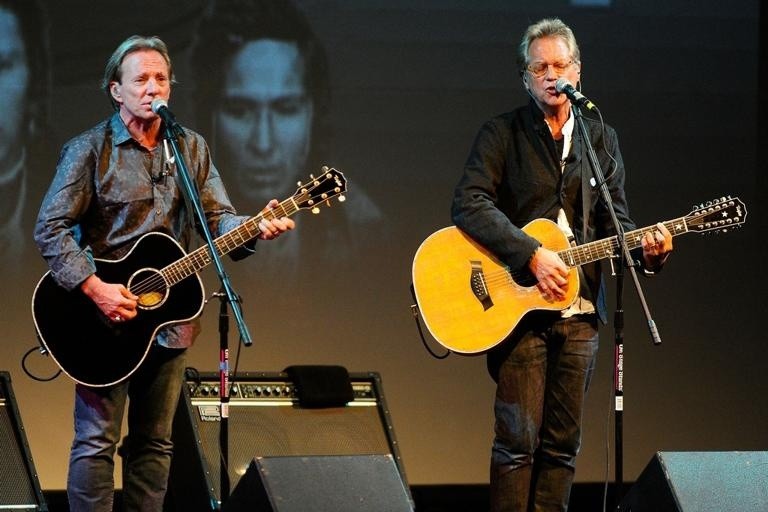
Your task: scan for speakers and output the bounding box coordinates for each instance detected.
[0,371,48,512]
[161,365,416,512]
[615,451,768,511]
[219,453,414,512]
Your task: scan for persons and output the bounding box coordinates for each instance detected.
[33,35,297,512]
[1,2,64,269]
[450,15,674,512]
[185,1,401,280]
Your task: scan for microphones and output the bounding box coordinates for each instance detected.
[554,77,600,115]
[151,97,186,139]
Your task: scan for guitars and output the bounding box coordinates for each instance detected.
[412,194,747,357]
[32,165,348,390]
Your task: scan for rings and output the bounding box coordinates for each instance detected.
[272,229,279,236]
[649,243,656,248]
[114,317,121,322]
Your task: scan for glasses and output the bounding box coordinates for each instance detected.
[526,58,575,78]
